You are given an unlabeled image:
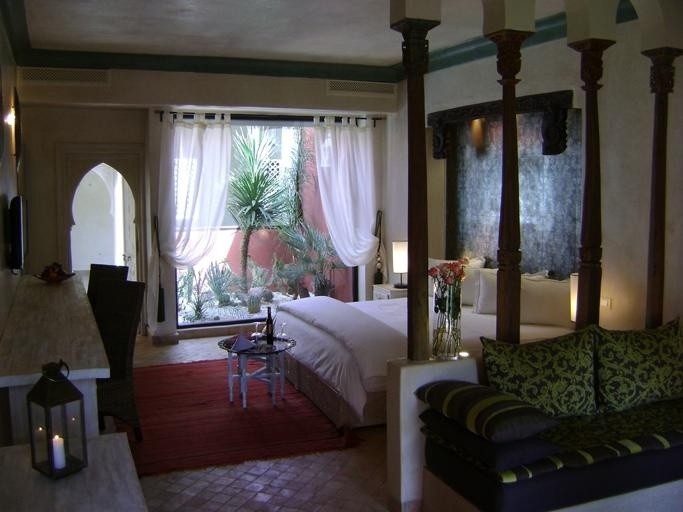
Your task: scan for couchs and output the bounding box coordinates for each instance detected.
[412,380,683,511]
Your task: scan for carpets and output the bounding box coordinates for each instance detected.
[114,353,360,480]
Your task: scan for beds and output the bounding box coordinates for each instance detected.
[263,295,594,433]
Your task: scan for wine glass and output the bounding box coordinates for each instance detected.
[276,320,288,350]
[250,322,262,345]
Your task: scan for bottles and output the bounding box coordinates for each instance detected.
[265,307,273,344]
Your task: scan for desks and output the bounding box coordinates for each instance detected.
[0,271,112,444]
[0,432,149,512]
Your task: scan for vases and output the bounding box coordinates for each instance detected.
[433,285,461,361]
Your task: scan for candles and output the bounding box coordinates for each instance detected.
[52,433,66,469]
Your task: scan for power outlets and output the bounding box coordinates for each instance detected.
[600,298,611,309]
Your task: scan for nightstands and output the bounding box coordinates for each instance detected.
[372,283,408,300]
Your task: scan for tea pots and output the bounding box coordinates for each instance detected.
[33,262,76,283]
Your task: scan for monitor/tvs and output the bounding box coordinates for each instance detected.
[5,195,28,270]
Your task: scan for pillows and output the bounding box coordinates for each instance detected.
[412,379,565,475]
[479,315,597,420]
[428,258,570,327]
[595,314,683,414]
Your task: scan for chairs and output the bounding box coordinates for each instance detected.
[86,263,146,442]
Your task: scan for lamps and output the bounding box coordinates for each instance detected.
[392,241,408,288]
[569,270,580,323]
